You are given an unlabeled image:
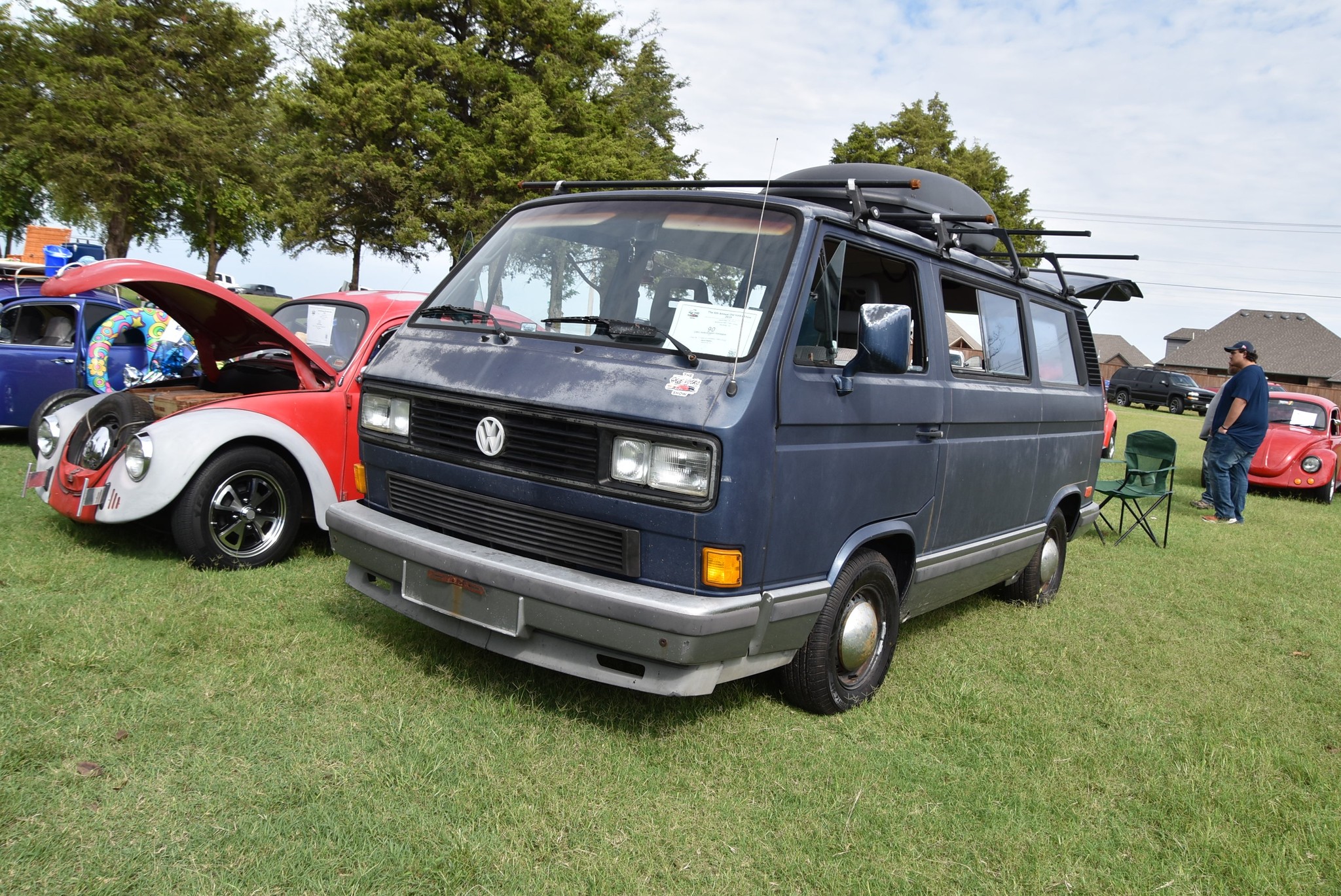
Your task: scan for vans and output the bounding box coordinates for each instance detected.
[325,162,1144,716]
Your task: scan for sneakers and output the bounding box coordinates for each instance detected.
[1190,500,1215,510]
[1201,513,1237,524]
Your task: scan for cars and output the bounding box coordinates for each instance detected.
[0,227,545,571]
[1247,391,1341,506]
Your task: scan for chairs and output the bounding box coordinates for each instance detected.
[795,343,856,366]
[650,276,710,328]
[32,316,73,346]
[1090,429,1179,549]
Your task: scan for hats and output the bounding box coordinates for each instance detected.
[1224,341,1254,353]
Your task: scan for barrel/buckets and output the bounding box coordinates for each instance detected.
[43,246,73,276]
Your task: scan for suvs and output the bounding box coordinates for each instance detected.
[1107,365,1217,414]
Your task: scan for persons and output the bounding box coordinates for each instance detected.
[1190,341,1269,524]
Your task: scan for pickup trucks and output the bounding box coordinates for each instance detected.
[1204,382,1294,422]
